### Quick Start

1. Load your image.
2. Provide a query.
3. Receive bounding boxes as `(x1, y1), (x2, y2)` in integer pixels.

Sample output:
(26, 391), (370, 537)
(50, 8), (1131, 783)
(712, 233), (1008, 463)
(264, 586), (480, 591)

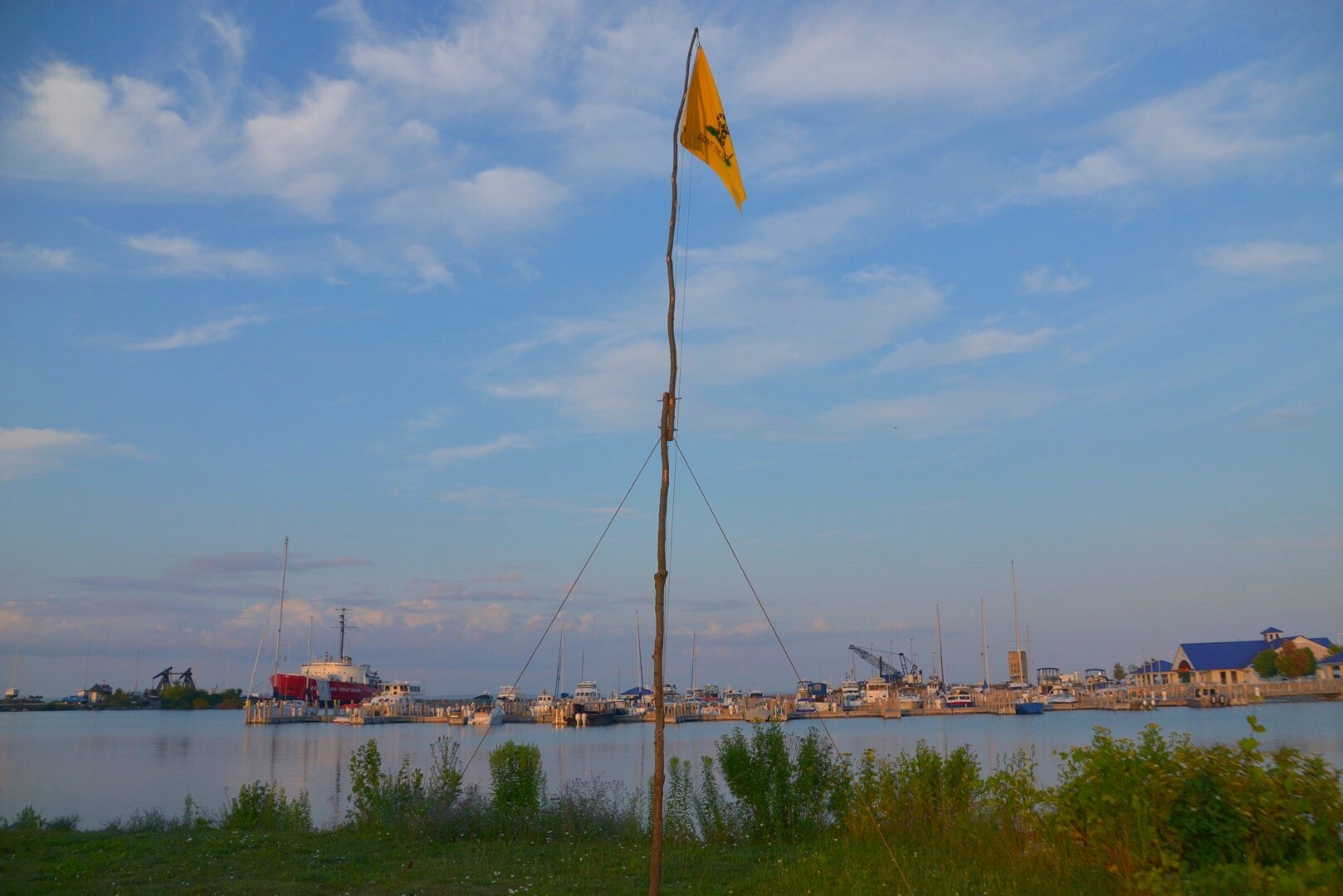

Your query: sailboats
(242, 537), (992, 728)
(1005, 558), (1046, 716)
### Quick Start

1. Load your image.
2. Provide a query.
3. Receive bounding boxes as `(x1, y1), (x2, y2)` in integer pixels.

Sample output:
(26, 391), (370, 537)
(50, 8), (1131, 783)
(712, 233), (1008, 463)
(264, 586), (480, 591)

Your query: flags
(680, 44), (746, 211)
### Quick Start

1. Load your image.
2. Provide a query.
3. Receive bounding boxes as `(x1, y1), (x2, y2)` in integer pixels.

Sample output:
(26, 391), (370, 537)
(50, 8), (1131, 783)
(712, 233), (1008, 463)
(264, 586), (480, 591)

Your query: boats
(1045, 688), (1078, 704)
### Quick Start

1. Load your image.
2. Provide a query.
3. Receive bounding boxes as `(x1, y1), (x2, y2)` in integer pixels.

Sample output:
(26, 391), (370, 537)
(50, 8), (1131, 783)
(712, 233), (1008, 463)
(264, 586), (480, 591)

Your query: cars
(60, 695), (88, 705)
